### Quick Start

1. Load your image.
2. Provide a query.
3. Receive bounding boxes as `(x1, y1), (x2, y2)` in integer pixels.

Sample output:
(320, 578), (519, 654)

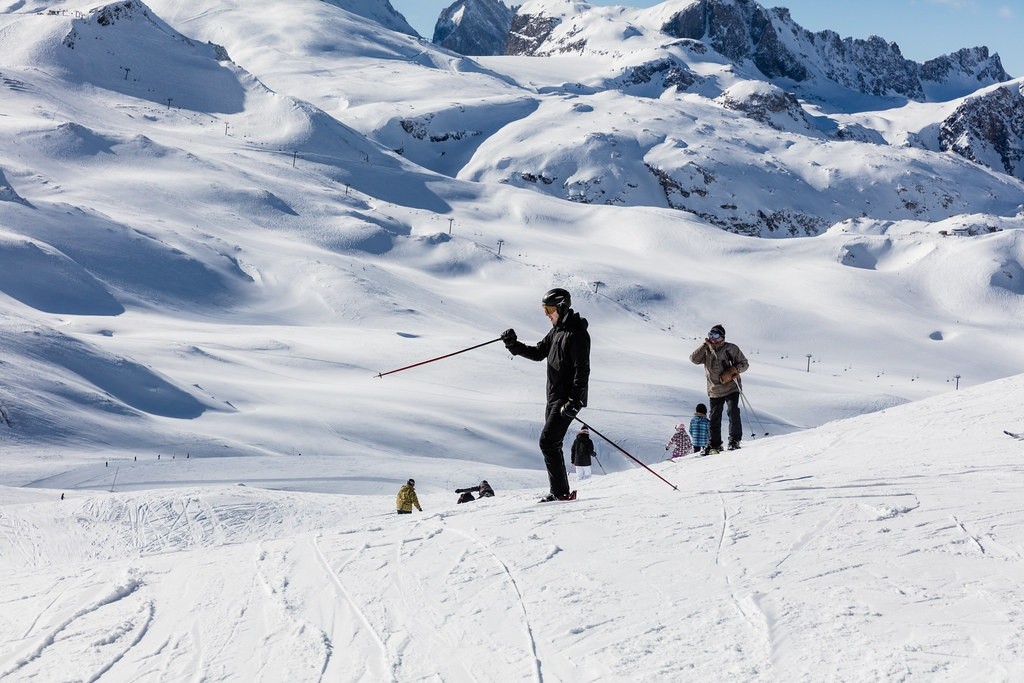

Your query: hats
(710, 325), (726, 338)
(696, 404), (707, 415)
(675, 423), (685, 430)
(581, 426), (588, 430)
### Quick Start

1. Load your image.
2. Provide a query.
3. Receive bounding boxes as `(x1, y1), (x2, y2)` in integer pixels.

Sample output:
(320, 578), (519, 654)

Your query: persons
(571, 425), (598, 480)
(689, 325), (749, 454)
(455, 480), (495, 500)
(665, 423), (693, 459)
(457, 491), (475, 504)
(396, 479), (423, 515)
(689, 403), (711, 453)
(500, 287), (592, 505)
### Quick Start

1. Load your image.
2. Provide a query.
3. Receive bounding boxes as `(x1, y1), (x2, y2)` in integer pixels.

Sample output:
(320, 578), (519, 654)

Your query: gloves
(592, 452), (596, 457)
(559, 401), (581, 420)
(571, 460), (574, 464)
(704, 338), (713, 349)
(501, 329), (518, 346)
(719, 366), (738, 385)
(666, 448), (668, 450)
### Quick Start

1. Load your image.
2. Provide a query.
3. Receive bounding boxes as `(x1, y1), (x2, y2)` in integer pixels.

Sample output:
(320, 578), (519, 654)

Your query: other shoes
(700, 443), (724, 455)
(540, 494), (569, 502)
(728, 439), (740, 450)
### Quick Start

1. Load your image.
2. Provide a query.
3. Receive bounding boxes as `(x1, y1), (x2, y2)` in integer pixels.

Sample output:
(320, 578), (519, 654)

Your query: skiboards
(665, 444), (748, 464)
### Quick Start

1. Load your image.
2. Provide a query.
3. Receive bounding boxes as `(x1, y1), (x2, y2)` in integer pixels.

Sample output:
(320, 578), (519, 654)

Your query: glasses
(708, 332), (720, 339)
(544, 306), (557, 314)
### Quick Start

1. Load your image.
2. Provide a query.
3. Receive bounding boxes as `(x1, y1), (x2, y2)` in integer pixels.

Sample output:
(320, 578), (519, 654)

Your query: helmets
(542, 288), (571, 307)
(406, 479), (415, 486)
